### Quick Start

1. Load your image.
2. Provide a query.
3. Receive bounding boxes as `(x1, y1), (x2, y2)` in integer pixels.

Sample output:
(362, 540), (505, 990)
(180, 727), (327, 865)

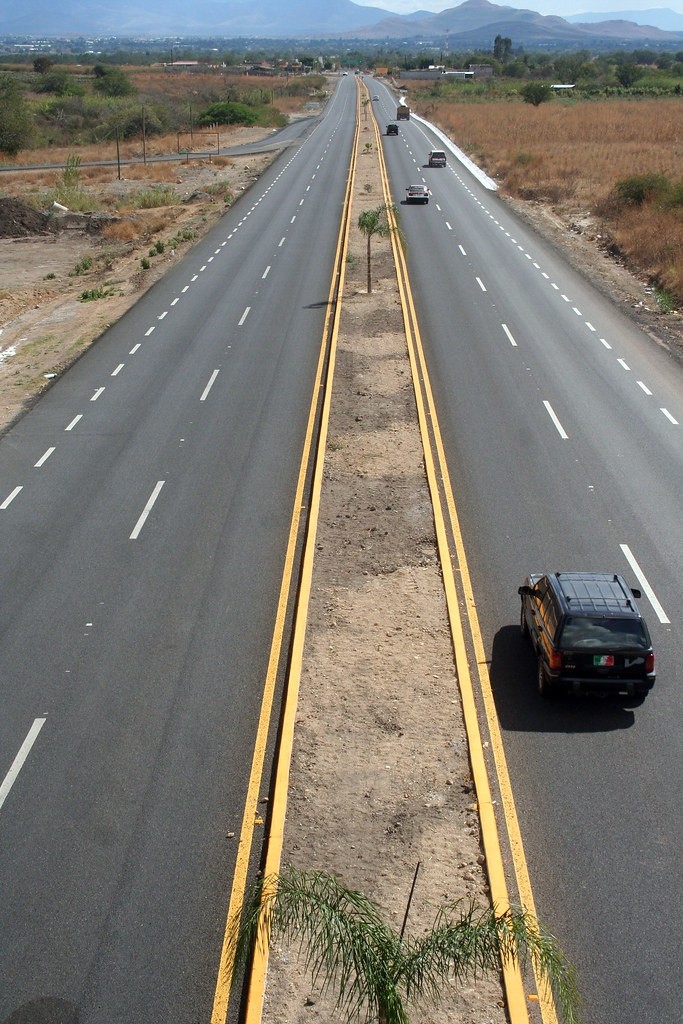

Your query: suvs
(518, 571), (656, 699)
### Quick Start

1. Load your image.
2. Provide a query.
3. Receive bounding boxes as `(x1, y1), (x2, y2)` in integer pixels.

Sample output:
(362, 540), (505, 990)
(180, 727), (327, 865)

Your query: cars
(405, 185), (430, 204)
(373, 95), (379, 101)
(386, 124), (399, 136)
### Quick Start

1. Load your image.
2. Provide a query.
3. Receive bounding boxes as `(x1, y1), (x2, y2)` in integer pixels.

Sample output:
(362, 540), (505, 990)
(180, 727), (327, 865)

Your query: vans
(428, 150), (447, 168)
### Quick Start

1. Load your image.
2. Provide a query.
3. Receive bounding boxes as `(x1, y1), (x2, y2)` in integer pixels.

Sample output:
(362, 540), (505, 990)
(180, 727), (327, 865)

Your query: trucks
(397, 105), (410, 120)
